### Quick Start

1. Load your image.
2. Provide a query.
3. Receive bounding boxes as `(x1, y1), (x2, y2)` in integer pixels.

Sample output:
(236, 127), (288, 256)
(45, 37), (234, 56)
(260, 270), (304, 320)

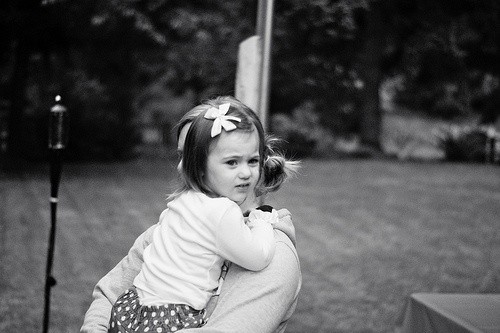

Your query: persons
(108, 95), (279, 332)
(79, 95), (303, 333)
